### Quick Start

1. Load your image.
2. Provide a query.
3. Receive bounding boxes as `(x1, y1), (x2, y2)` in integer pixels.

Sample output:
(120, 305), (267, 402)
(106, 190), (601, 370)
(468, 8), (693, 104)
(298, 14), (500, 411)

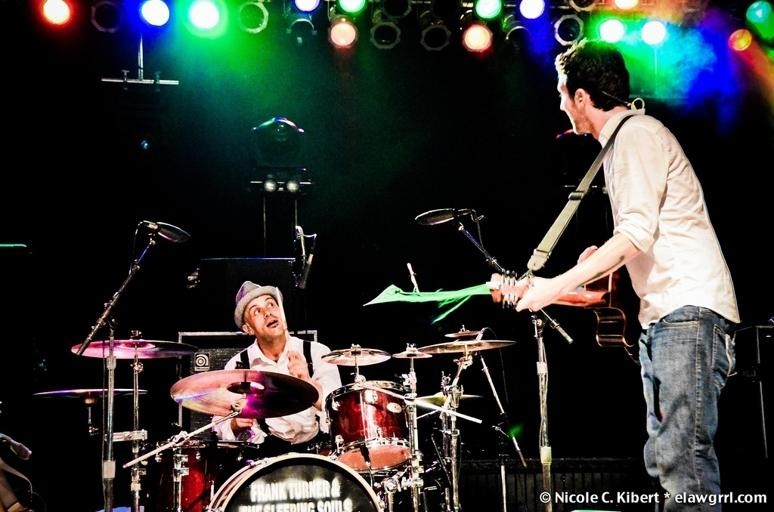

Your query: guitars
(489, 244), (643, 365)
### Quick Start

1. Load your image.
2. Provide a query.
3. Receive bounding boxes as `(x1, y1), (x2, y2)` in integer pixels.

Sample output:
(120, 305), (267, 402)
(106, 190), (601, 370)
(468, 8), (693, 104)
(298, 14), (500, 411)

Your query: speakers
(716, 319), (774, 512)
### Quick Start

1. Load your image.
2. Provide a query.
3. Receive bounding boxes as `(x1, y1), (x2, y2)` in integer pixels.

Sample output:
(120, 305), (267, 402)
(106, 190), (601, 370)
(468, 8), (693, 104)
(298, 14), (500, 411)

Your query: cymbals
(70, 338), (198, 360)
(170, 369), (319, 417)
(418, 340), (517, 354)
(444, 330), (479, 338)
(392, 350), (433, 360)
(321, 348), (393, 367)
(33, 387), (149, 401)
(415, 391), (483, 405)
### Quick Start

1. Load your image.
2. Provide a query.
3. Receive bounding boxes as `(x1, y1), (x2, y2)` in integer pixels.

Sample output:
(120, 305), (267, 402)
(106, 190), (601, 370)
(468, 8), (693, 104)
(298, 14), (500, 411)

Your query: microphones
(0, 432), (33, 461)
(415, 207), (471, 227)
(154, 221), (193, 244)
(294, 252), (314, 291)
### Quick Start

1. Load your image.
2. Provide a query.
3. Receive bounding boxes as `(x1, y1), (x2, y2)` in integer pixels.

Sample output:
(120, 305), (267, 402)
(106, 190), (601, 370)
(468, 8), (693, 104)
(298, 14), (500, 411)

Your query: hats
(235, 280), (283, 328)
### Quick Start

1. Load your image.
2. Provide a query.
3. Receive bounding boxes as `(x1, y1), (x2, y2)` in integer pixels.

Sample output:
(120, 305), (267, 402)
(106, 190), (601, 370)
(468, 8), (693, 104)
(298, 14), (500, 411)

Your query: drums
(207, 452), (386, 512)
(325, 380), (412, 473)
(169, 442), (257, 512)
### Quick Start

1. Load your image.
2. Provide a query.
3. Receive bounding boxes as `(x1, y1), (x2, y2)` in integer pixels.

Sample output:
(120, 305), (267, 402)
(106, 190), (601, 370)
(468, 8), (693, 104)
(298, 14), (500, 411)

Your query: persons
(207, 277), (347, 495)
(512, 35), (740, 511)
